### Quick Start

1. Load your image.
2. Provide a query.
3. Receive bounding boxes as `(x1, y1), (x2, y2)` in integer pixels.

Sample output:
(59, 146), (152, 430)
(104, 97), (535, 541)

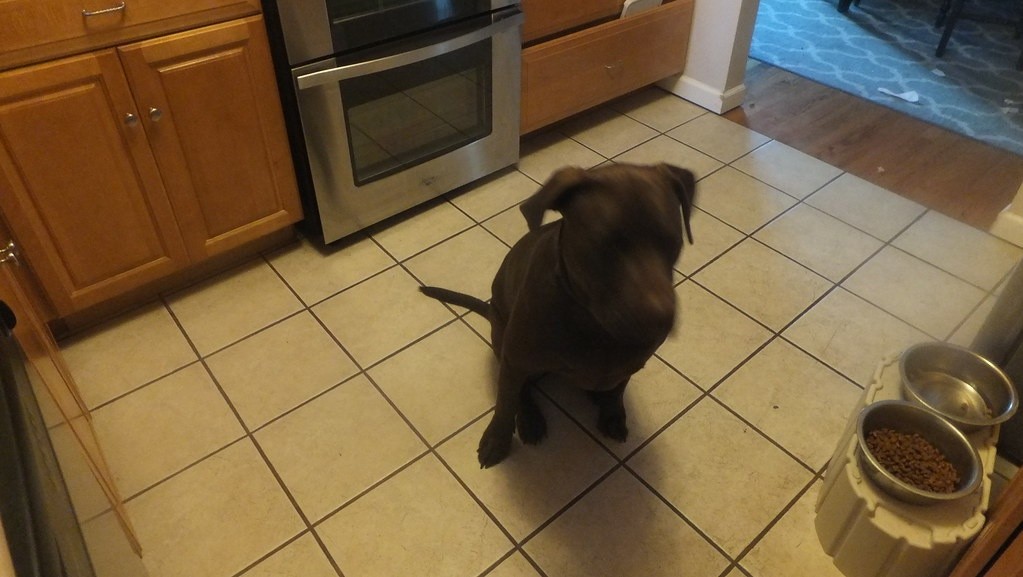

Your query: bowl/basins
(900, 343), (1018, 432)
(855, 400), (983, 502)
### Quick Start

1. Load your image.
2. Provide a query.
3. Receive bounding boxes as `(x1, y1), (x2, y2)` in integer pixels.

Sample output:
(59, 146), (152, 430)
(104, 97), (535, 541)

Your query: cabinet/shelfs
(519, 0), (694, 135)
(0, 0), (305, 325)
(1, 244), (154, 577)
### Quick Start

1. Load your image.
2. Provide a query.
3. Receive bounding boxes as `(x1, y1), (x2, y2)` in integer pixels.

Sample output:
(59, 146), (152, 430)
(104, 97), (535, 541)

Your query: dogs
(417, 162), (699, 470)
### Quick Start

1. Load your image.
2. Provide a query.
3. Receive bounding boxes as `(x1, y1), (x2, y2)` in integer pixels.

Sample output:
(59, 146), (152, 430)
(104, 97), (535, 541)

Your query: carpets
(747, 0), (1023, 160)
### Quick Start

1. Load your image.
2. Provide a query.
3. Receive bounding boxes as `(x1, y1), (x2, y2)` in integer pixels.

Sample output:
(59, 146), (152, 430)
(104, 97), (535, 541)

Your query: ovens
(259, 0), (521, 247)
